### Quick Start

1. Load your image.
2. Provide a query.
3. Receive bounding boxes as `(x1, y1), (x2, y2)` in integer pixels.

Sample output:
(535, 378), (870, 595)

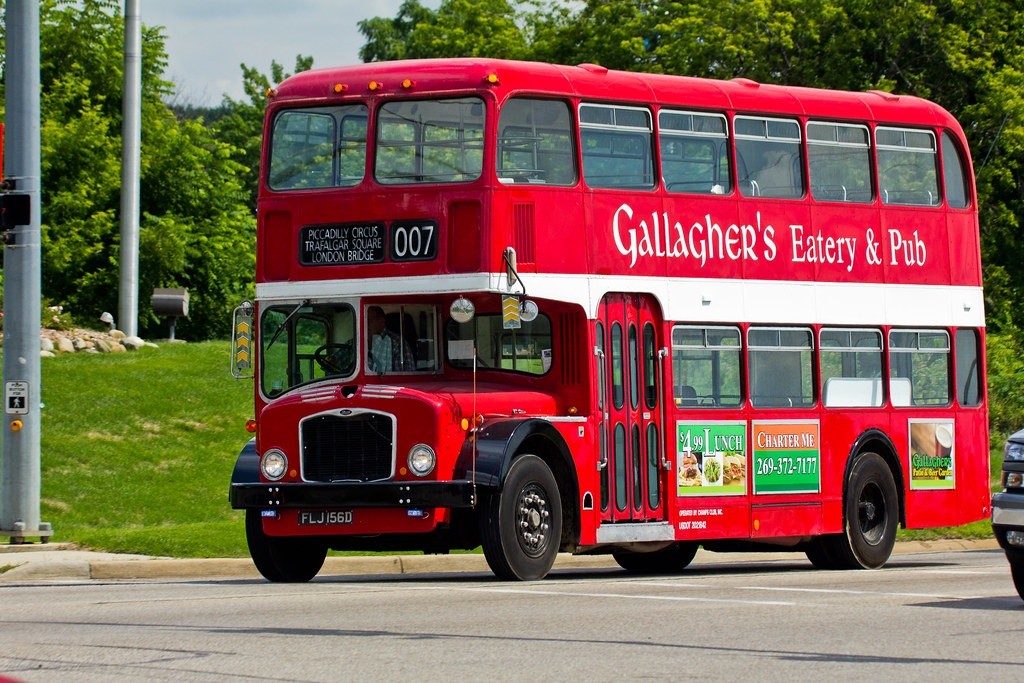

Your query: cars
(990, 425), (1024, 600)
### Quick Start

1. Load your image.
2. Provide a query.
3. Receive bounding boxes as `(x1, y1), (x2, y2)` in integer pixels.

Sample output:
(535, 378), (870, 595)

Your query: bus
(229, 55), (994, 573)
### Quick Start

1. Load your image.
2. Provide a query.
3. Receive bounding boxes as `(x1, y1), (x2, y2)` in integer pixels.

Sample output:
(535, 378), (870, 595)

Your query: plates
(701, 451), (724, 488)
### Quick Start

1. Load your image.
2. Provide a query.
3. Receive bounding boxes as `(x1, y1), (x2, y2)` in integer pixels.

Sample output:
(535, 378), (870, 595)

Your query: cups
(934, 426), (952, 480)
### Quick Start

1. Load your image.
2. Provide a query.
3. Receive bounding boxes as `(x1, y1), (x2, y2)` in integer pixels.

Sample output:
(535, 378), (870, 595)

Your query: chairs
(323, 172), (943, 408)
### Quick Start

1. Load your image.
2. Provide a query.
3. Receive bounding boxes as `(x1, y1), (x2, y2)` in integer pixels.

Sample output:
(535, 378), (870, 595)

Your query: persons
(320, 305), (416, 374)
